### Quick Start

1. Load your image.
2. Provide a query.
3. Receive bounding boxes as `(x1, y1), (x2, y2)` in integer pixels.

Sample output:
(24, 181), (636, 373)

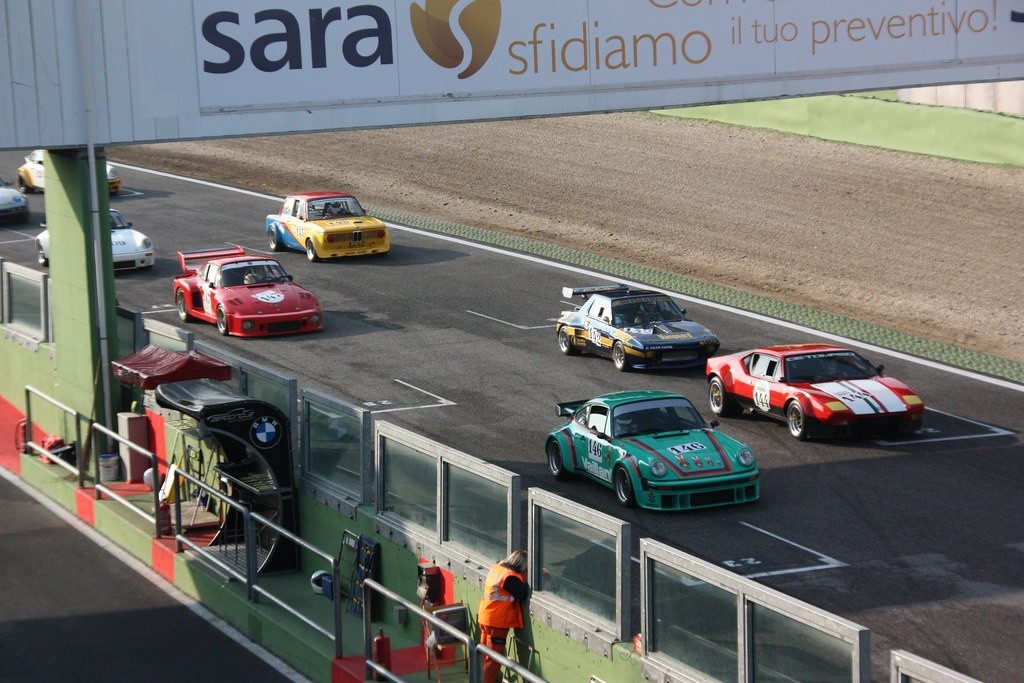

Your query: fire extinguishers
(159, 499), (171, 535)
(374, 627), (391, 681)
(14, 418), (32, 453)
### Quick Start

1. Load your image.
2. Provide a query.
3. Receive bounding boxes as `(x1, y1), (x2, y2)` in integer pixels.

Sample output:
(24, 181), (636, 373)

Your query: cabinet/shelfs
(117, 412), (149, 484)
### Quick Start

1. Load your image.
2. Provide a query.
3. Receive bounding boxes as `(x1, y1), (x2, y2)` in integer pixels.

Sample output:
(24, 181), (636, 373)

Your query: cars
(0, 179), (28, 226)
(554, 282), (721, 372)
(34, 208), (155, 275)
(15, 148), (120, 197)
(265, 190), (391, 262)
(172, 246), (325, 338)
(704, 343), (925, 441)
(543, 389), (762, 514)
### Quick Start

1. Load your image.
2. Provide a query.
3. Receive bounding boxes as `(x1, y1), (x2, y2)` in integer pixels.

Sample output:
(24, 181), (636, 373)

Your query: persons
(325, 201), (349, 219)
(476, 549), (530, 683)
(244, 266), (276, 286)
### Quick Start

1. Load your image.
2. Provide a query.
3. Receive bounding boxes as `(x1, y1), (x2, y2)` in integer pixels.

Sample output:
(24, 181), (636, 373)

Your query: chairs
(422, 599), (469, 683)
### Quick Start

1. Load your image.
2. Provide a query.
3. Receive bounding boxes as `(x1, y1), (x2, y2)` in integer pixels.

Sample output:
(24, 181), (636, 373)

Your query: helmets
(329, 201), (341, 212)
(311, 570), (332, 594)
(821, 357), (839, 370)
(640, 301), (656, 312)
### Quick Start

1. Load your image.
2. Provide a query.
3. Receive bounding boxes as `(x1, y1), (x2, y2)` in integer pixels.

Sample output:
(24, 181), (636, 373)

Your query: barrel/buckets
(98, 454), (118, 481)
